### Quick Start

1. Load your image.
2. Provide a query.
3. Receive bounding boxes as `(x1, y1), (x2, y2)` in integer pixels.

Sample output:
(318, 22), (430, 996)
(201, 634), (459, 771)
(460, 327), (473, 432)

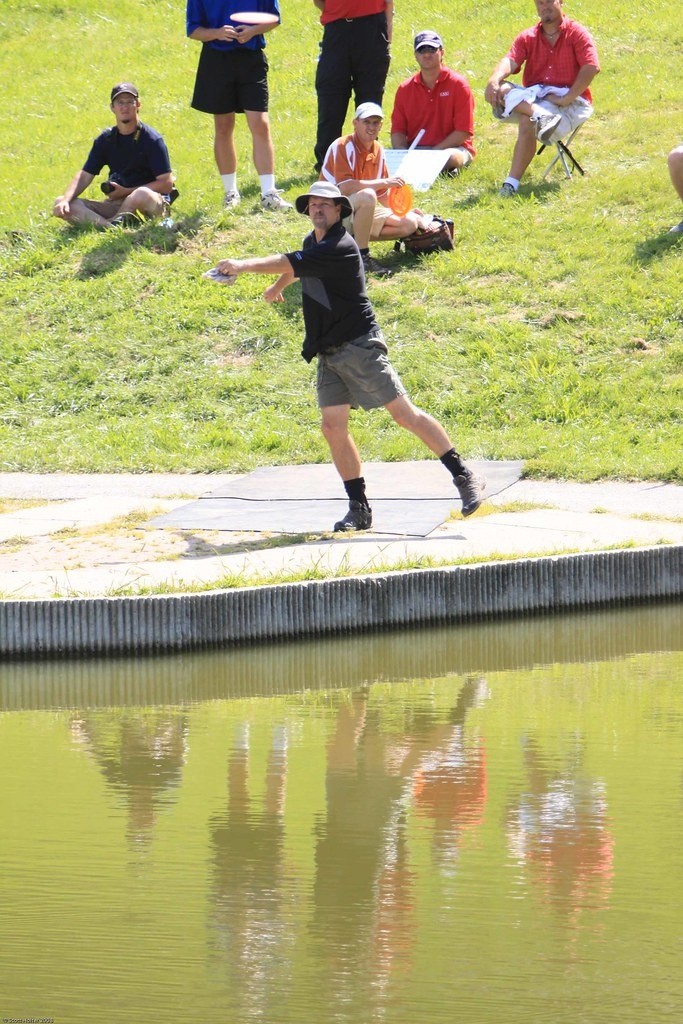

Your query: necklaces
(543, 29), (559, 38)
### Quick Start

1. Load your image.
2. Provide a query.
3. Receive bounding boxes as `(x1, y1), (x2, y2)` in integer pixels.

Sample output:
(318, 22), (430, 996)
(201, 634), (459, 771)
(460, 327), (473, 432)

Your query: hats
(110, 82), (139, 102)
(294, 182), (353, 220)
(414, 31), (443, 52)
(354, 102), (383, 120)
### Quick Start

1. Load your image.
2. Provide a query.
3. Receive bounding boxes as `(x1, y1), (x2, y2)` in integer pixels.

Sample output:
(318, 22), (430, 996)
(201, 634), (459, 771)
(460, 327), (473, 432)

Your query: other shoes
(448, 167), (460, 178)
(670, 219), (683, 233)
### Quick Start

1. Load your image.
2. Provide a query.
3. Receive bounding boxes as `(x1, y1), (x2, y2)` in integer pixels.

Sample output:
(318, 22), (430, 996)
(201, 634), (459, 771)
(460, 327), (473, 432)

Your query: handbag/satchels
(405, 215), (456, 256)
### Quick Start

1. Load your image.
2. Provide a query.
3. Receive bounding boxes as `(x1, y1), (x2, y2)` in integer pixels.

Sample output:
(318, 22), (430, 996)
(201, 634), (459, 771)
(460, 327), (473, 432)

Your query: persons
(313, 0), (393, 173)
(485, 0), (601, 198)
(391, 31), (476, 174)
(185, 0), (295, 214)
(318, 102), (424, 277)
(53, 83), (175, 233)
(215, 182), (487, 533)
(667, 144), (683, 232)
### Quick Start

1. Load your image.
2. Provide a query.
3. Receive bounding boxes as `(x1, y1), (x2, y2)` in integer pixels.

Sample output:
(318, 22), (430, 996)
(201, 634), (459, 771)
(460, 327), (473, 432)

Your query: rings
(224, 269), (228, 273)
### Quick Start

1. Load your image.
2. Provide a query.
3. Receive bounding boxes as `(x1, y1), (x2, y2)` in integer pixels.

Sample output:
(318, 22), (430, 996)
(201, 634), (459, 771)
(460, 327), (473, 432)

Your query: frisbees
(229, 12), (278, 25)
(388, 184), (412, 216)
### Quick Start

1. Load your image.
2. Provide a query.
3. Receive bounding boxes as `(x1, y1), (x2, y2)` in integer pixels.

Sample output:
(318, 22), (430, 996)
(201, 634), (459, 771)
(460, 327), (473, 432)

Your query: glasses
(417, 45), (438, 54)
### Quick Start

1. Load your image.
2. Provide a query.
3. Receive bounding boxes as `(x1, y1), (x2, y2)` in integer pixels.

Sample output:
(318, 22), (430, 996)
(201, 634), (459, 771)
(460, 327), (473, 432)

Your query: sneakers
(529, 113), (563, 143)
(334, 500), (372, 532)
(453, 470), (487, 517)
(497, 183), (515, 199)
(223, 191), (241, 213)
(361, 254), (391, 279)
(259, 189), (293, 213)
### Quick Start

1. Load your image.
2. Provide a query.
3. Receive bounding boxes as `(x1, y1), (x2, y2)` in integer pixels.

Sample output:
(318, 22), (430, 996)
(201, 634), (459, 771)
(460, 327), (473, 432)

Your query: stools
(542, 123), (583, 181)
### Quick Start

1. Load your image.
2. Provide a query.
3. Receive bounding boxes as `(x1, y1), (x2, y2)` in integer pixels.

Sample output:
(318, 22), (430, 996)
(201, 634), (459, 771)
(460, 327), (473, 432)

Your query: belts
(321, 343), (343, 355)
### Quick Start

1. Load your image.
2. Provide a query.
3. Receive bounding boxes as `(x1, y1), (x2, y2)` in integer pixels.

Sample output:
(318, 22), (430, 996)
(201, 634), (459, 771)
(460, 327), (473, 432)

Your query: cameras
(100, 172), (124, 194)
